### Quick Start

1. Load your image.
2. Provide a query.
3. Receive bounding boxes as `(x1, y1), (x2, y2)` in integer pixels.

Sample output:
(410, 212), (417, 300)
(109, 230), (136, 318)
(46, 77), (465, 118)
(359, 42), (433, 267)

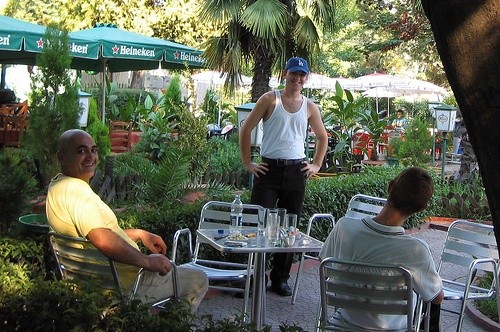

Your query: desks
(110, 131), (178, 153)
(196, 226), (325, 332)
(0, 127), (25, 149)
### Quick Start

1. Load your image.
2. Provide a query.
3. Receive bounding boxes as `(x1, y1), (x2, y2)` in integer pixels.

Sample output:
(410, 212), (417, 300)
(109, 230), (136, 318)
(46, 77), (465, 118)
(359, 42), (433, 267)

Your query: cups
(257, 207), (297, 243)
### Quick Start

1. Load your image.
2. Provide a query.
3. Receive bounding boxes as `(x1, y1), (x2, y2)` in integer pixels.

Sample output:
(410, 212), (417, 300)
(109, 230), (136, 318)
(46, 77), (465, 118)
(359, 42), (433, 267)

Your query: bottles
(229, 195), (243, 234)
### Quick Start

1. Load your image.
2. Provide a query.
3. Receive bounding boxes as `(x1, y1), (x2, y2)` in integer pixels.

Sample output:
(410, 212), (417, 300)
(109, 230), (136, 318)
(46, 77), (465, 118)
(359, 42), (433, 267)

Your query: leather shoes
(272, 281), (292, 296)
(235, 284), (267, 298)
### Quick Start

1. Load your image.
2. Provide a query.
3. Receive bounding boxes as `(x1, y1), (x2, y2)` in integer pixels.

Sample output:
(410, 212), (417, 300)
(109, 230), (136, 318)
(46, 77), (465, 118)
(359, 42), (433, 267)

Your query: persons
(319, 166), (444, 332)
(45, 128), (209, 324)
(234, 57), (328, 299)
(390, 109), (409, 139)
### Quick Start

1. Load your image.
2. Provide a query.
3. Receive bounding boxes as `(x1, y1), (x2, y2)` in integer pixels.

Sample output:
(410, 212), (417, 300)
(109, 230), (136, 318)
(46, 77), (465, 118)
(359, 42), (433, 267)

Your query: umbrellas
(190, 72), (449, 128)
(0, 15), (209, 126)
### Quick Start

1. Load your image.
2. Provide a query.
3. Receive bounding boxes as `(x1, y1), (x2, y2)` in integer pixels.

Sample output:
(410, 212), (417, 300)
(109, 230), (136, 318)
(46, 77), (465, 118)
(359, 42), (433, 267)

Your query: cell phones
(224, 241), (248, 246)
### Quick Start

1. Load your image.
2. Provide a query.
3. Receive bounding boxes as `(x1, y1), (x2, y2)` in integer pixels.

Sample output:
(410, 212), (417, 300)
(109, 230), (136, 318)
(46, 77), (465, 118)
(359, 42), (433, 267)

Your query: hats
(0, 89), (16, 104)
(285, 57), (309, 75)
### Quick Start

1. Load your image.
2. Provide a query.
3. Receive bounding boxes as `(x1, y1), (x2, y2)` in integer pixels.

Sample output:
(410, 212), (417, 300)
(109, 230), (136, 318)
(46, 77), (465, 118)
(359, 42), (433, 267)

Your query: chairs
(2, 100), (28, 147)
(19, 193), (500, 332)
(207, 119), (235, 141)
(109, 120), (132, 153)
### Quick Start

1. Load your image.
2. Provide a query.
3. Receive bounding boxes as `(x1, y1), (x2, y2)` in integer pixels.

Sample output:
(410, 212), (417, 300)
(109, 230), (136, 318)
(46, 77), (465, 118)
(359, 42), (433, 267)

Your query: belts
(261, 157), (305, 167)
